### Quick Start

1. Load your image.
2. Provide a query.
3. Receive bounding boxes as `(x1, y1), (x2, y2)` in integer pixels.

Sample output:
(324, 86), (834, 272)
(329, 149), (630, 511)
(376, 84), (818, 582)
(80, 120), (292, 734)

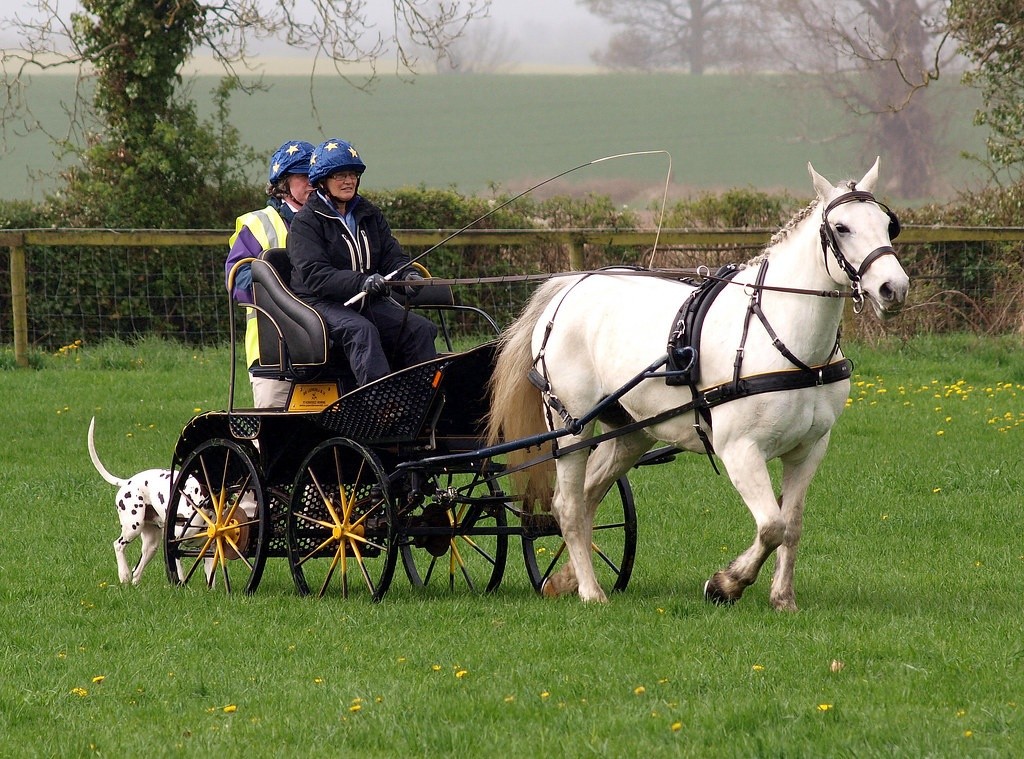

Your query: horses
(472, 156), (911, 611)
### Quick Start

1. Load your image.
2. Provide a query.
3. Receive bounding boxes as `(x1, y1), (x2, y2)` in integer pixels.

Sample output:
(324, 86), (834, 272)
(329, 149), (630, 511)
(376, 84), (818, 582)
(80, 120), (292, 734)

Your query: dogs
(88, 416), (256, 591)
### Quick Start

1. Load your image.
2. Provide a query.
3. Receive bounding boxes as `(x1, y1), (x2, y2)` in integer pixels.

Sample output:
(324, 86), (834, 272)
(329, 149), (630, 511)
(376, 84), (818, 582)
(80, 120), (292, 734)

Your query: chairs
(250, 246), (455, 378)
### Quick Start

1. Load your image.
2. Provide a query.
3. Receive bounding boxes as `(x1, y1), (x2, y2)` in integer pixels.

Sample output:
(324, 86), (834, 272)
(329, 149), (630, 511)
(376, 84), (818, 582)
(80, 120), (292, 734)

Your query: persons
(224, 141), (318, 548)
(286, 138), (437, 436)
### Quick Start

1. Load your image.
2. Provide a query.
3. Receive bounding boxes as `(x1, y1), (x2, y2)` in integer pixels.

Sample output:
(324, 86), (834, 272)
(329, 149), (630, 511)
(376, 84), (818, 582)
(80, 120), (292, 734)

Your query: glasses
(327, 173), (360, 181)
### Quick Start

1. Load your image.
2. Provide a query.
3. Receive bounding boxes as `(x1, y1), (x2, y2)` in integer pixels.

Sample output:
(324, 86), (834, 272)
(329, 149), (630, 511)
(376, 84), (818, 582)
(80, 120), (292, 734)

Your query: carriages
(164, 148), (918, 612)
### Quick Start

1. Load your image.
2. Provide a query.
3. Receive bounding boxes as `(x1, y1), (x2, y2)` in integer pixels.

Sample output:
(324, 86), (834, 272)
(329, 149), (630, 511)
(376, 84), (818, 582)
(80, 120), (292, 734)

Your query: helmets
(309, 138), (367, 190)
(269, 140), (316, 182)
